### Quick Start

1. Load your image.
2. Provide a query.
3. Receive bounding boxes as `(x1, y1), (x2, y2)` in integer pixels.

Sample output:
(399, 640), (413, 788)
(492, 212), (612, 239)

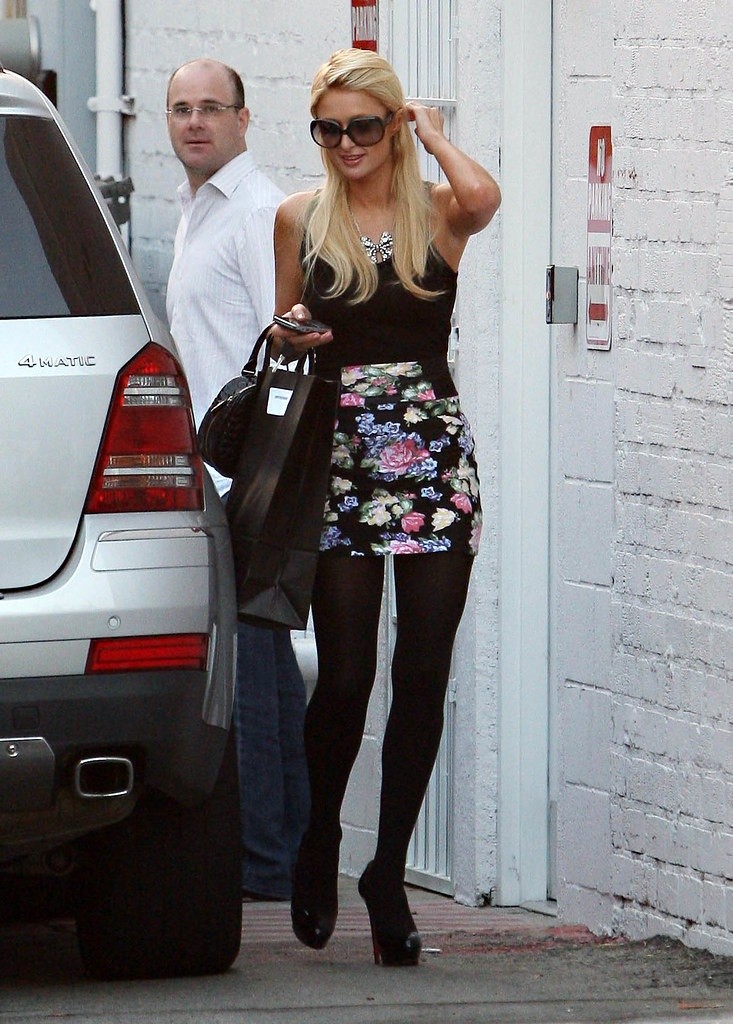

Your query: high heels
(291, 827), (342, 949)
(358, 860), (421, 966)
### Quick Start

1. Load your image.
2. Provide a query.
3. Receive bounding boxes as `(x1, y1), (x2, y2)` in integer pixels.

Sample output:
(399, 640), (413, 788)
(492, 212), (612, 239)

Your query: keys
(272, 340), (296, 374)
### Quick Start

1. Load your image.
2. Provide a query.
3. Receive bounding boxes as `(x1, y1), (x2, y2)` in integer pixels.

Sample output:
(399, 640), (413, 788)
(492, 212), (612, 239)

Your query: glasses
(310, 112), (393, 148)
(165, 104), (240, 118)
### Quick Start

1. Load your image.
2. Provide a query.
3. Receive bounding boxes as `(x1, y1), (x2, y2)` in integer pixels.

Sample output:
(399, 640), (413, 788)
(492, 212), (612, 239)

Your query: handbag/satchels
(226, 327), (342, 632)
(197, 322), (315, 479)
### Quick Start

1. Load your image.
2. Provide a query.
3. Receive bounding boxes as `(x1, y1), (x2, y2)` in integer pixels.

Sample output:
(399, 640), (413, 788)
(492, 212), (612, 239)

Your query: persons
(164, 59), (307, 903)
(197, 48), (501, 965)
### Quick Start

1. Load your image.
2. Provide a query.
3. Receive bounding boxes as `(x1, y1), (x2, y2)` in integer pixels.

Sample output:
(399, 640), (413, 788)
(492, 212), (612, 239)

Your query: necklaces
(349, 200), (397, 263)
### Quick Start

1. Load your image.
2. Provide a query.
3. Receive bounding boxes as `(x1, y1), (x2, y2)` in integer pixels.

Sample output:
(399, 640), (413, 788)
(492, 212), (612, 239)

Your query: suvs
(1, 71), (249, 986)
